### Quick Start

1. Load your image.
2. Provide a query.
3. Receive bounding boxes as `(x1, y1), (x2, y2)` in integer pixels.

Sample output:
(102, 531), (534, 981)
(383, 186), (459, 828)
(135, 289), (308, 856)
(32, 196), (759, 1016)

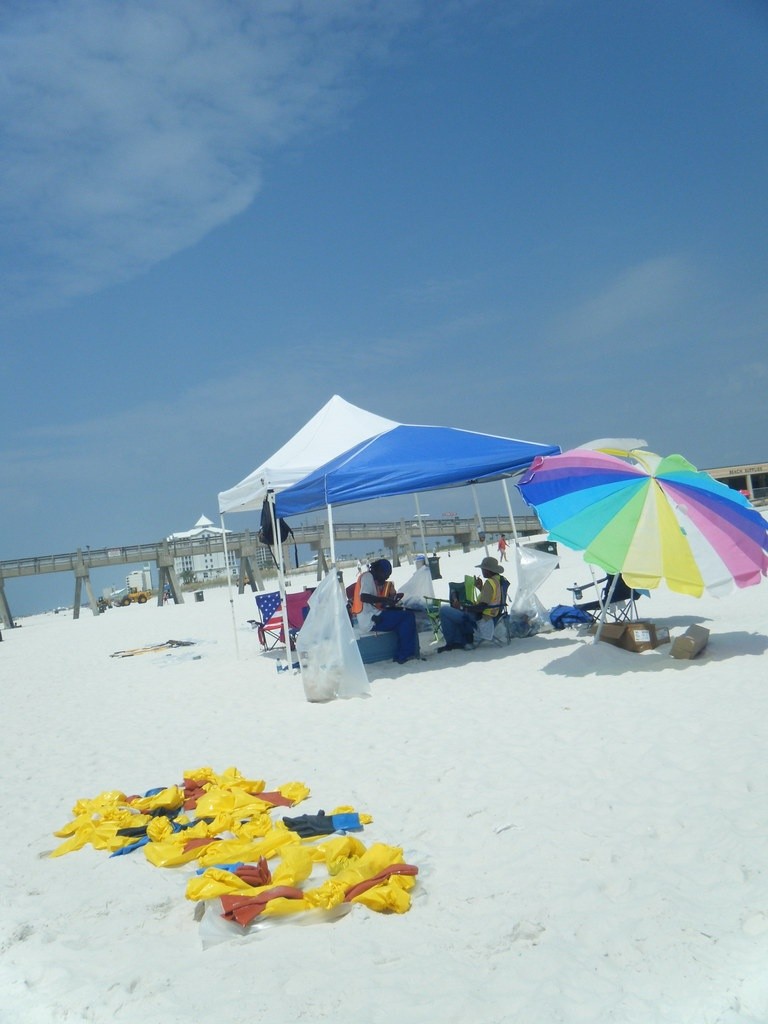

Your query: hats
(475, 557), (504, 573)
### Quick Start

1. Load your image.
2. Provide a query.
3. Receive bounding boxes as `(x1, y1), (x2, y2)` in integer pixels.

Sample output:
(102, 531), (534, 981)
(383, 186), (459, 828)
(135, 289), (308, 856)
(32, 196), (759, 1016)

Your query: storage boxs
(587, 622), (658, 654)
(668, 623), (710, 659)
(655, 627), (671, 645)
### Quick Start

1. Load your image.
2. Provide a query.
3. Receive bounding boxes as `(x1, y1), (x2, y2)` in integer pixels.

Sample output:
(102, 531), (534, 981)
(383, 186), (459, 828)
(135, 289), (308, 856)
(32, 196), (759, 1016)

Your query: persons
(436, 556), (504, 654)
(433, 545), (436, 556)
(366, 557), (370, 572)
(354, 558), (363, 577)
(352, 558), (426, 665)
(497, 534), (510, 562)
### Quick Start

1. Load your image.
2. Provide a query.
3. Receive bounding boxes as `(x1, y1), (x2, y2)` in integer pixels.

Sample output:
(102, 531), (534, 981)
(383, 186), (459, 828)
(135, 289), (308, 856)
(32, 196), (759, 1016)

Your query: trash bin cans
(415, 556), (443, 580)
(194, 591), (204, 603)
(523, 541), (559, 569)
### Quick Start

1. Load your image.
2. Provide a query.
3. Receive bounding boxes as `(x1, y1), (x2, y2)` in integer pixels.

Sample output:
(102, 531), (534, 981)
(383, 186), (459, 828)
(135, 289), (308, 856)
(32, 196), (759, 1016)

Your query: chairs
(469, 575), (510, 649)
(255, 592), (290, 652)
(285, 591), (312, 642)
(422, 575), (476, 645)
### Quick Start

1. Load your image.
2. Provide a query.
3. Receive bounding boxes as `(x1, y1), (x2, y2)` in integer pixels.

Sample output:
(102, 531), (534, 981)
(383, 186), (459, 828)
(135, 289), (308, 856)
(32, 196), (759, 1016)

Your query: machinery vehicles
(109, 586), (151, 606)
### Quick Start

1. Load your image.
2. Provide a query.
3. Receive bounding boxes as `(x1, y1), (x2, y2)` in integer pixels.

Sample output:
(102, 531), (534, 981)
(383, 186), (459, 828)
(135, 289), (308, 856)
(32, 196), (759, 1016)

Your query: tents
(213, 393), (562, 680)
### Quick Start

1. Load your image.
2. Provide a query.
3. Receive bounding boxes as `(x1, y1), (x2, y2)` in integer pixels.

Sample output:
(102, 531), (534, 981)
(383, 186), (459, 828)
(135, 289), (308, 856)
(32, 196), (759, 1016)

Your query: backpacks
(549, 604), (596, 630)
(257, 490), (301, 572)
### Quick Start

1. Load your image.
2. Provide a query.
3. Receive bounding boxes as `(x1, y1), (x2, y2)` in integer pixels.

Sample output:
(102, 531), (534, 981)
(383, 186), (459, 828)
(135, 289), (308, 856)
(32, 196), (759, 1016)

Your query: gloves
(47, 762), (418, 933)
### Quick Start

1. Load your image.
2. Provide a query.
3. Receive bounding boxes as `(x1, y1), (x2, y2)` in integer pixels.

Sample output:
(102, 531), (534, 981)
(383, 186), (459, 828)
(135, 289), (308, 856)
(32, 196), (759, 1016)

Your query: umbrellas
(513, 436), (767, 646)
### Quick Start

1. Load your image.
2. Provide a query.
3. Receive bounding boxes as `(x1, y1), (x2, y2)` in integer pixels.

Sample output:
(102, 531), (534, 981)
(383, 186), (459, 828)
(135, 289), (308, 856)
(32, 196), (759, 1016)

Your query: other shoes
(393, 655), (427, 664)
(437, 642), (466, 653)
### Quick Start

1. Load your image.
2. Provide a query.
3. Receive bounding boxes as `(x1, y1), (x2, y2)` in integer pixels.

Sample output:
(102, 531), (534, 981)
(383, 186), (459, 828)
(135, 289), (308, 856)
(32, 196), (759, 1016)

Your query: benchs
(574, 573), (640, 629)
(353, 630), (418, 664)
